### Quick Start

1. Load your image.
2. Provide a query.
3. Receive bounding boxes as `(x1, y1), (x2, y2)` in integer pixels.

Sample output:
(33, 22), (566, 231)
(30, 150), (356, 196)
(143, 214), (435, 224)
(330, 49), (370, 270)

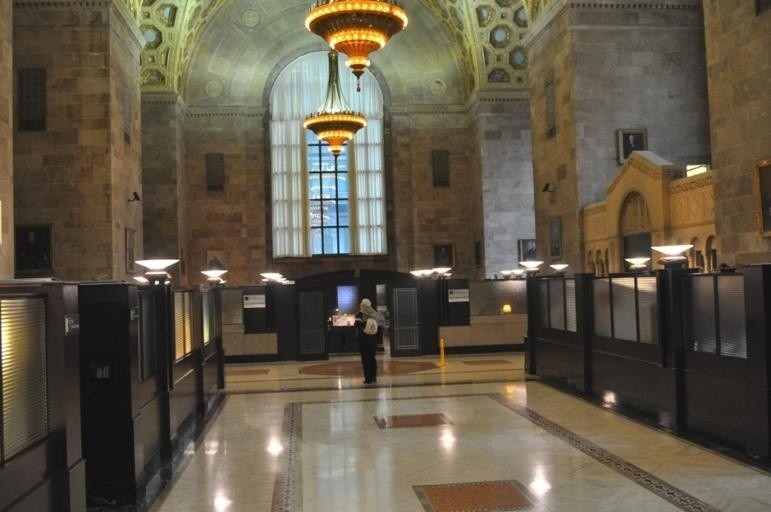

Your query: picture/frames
(750, 158), (771, 238)
(204, 247), (228, 271)
(517, 238), (538, 264)
(432, 242), (457, 269)
(546, 215), (564, 261)
(124, 225), (137, 275)
(615, 127), (648, 166)
(14, 222), (54, 276)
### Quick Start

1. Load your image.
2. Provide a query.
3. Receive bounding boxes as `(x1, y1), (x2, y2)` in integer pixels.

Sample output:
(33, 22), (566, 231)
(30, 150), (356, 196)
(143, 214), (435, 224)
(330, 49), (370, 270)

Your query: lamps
(297, 2), (410, 168)
(500, 262), (569, 281)
(409, 267), (452, 281)
(494, 302), (512, 315)
(135, 246), (230, 282)
(257, 269), (296, 286)
(541, 183), (555, 193)
(625, 239), (694, 267)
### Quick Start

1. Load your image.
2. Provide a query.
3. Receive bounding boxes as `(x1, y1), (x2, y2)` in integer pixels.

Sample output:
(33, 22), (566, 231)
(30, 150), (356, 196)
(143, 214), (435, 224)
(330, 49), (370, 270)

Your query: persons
(624, 134), (641, 159)
(354, 296), (386, 385)
(17, 230), (47, 269)
(528, 242), (536, 258)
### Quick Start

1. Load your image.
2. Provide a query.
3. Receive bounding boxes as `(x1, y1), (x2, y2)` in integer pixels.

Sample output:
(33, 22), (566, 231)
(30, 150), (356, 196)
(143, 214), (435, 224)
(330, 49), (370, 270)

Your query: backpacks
(355, 318), (378, 335)
